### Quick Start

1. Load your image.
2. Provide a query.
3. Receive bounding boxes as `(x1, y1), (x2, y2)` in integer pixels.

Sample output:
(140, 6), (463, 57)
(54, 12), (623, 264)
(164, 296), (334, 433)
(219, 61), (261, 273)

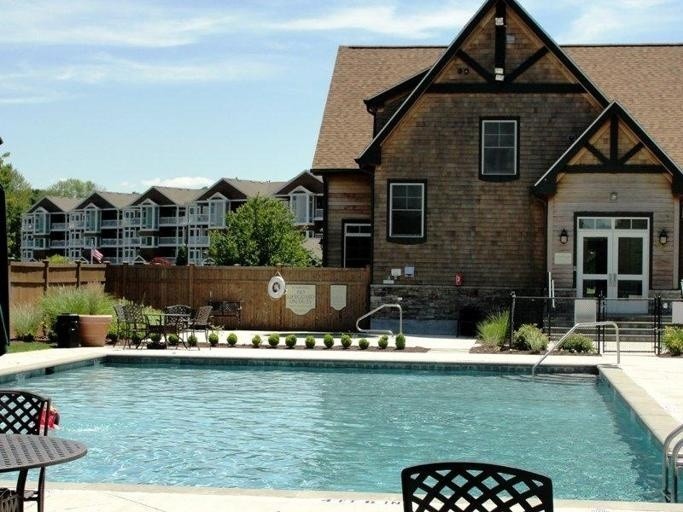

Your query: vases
(78, 314), (113, 346)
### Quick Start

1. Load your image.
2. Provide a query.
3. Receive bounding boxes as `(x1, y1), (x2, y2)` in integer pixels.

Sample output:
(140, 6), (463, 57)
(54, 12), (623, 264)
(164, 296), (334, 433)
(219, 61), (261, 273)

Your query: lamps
(659, 229), (667, 245)
(560, 228), (568, 244)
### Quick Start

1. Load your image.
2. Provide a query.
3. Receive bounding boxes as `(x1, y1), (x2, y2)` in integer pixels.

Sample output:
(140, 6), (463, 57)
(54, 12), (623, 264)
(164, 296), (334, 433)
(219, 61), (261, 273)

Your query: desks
(0, 433), (88, 512)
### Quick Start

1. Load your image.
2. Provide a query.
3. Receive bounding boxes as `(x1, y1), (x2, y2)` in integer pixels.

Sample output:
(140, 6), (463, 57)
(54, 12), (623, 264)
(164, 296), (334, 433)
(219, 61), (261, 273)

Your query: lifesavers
(267, 277), (285, 298)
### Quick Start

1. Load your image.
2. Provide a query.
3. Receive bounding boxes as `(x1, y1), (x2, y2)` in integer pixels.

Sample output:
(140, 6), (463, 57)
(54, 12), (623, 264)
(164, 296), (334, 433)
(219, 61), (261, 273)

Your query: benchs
(209, 301), (241, 330)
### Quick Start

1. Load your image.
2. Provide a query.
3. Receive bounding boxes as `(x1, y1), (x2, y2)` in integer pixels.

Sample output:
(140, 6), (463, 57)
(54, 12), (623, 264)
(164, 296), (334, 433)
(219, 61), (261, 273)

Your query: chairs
(401, 460), (553, 512)
(113, 303), (212, 350)
(0, 389), (51, 512)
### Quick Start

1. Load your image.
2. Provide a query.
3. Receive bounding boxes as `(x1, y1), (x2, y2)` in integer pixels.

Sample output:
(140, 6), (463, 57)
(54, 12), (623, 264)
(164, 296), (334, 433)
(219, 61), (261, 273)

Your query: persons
(36, 404), (60, 428)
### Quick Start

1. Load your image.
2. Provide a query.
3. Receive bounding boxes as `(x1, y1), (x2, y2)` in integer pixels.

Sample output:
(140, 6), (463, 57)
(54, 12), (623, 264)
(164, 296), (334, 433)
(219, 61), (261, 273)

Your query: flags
(92, 247), (103, 263)
